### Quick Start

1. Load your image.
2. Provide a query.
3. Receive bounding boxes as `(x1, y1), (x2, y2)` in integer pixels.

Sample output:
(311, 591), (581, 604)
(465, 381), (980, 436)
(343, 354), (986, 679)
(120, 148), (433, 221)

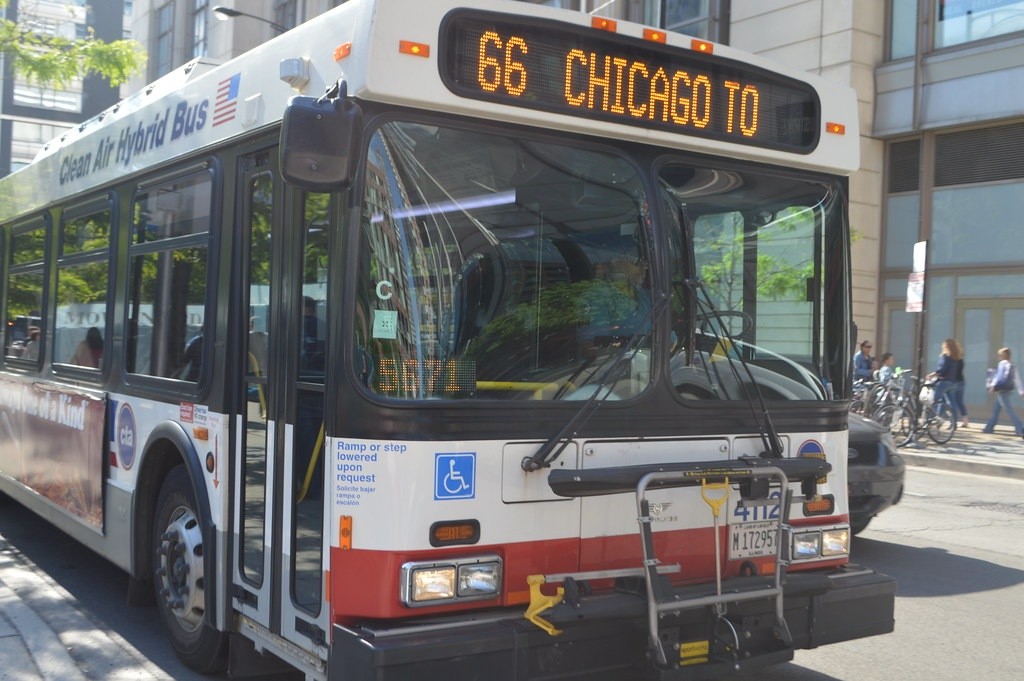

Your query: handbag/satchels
(918, 379), (935, 404)
(985, 364), (1015, 391)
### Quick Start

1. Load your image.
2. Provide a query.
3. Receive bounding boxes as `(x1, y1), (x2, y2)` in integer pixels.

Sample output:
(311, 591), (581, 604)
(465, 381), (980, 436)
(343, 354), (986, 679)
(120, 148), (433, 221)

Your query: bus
(0, 0), (896, 681)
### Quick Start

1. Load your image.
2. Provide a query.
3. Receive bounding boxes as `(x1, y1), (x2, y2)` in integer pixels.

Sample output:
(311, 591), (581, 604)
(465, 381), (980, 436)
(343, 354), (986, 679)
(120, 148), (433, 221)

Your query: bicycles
(849, 368), (957, 448)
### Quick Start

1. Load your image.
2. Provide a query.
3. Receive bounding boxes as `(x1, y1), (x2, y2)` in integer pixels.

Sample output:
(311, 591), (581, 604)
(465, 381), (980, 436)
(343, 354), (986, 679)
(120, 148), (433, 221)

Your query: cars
(848, 412), (907, 536)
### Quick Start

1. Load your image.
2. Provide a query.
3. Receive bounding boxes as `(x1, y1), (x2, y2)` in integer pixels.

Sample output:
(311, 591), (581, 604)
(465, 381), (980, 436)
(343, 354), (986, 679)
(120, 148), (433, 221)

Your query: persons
(982, 347), (1024, 436)
(574, 232), (679, 357)
(944, 343), (969, 430)
(877, 353), (901, 432)
(69, 326), (105, 370)
(15, 324), (50, 361)
(923, 338), (960, 432)
(176, 292), (329, 390)
(853, 340), (875, 394)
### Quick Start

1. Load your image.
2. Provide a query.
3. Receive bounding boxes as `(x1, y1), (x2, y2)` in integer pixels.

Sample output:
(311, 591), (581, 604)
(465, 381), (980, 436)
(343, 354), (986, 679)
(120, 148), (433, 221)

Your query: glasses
(864, 346), (872, 348)
(623, 254), (649, 269)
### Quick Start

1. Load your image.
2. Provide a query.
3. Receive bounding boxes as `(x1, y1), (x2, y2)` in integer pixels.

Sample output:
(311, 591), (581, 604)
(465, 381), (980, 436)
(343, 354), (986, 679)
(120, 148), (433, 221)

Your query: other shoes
(946, 423), (957, 429)
(960, 424), (969, 428)
